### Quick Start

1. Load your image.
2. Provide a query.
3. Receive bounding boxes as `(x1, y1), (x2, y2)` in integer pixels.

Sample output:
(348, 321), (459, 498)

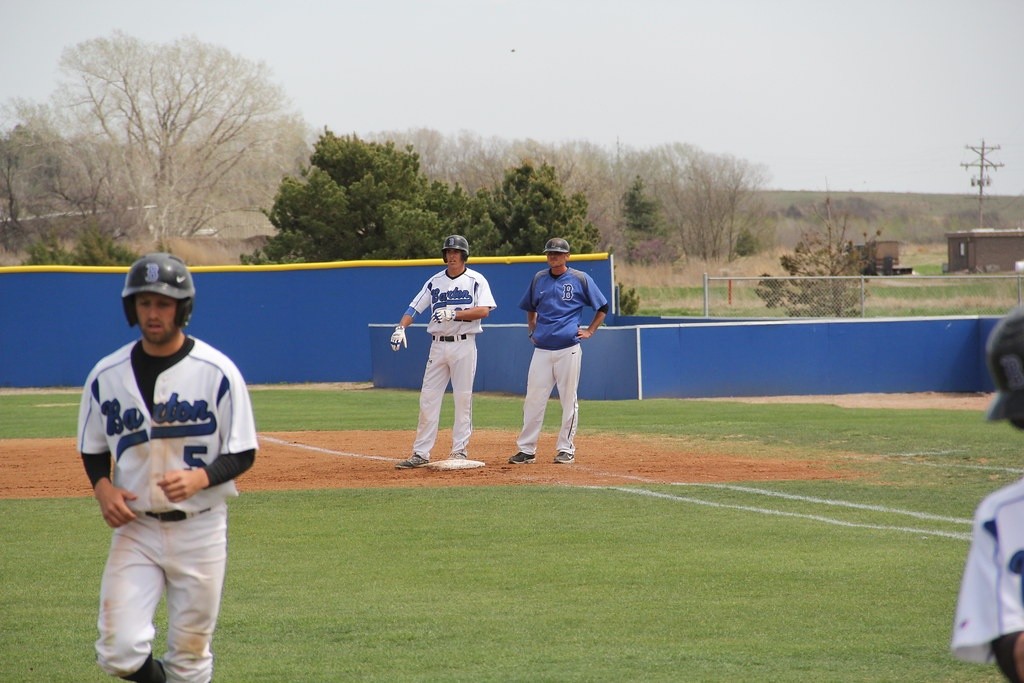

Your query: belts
(145, 509), (211, 521)
(432, 334), (467, 341)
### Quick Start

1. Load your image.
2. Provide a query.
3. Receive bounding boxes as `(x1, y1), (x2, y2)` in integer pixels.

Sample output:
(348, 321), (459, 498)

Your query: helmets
(441, 235), (469, 261)
(541, 238), (571, 253)
(120, 254), (195, 329)
(987, 302), (1023, 424)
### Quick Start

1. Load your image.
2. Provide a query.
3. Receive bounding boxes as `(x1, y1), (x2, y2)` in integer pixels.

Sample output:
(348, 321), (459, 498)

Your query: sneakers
(395, 455), (429, 468)
(447, 453), (466, 460)
(554, 451), (575, 464)
(508, 452), (536, 464)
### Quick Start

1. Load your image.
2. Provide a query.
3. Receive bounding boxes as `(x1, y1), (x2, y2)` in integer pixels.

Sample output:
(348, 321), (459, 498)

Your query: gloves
(431, 308), (456, 324)
(390, 325), (408, 351)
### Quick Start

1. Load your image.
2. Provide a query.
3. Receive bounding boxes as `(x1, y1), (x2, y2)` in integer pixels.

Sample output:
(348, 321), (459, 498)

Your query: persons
(508, 238), (609, 464)
(75, 252), (259, 683)
(951, 307), (1024, 683)
(391, 235), (497, 469)
